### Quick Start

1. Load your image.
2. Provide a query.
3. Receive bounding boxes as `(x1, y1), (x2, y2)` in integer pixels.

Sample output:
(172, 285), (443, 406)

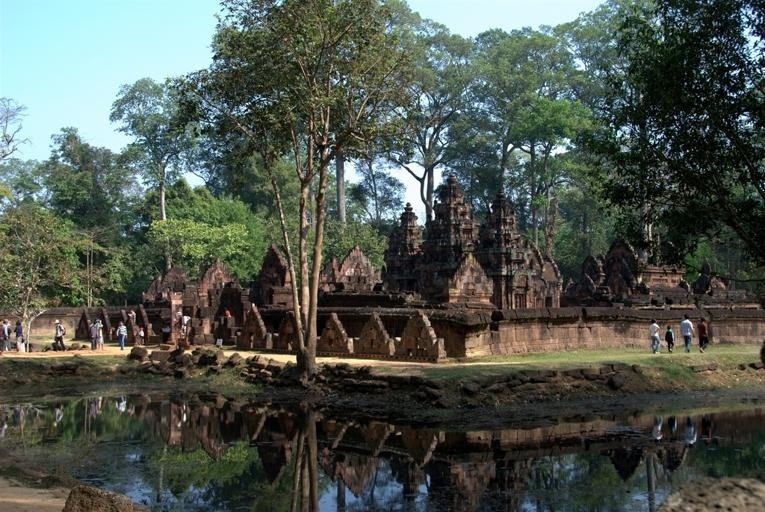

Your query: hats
(177, 312), (182, 315)
(54, 319), (60, 324)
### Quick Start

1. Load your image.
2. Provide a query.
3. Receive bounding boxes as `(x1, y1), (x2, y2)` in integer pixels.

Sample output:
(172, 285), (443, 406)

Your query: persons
(174, 311), (191, 339)
(665, 324), (675, 353)
(685, 415), (698, 449)
(701, 414), (712, 449)
(51, 402), (64, 428)
(139, 326), (145, 344)
(0, 402), (25, 439)
(680, 313), (696, 352)
(668, 415), (678, 444)
(649, 318), (661, 354)
(89, 317), (105, 351)
(697, 317), (709, 353)
(87, 394), (135, 420)
(52, 319), (67, 351)
(651, 414), (664, 446)
(162, 325), (171, 341)
(175, 402), (188, 427)
(0, 318), (26, 353)
(116, 321), (127, 350)
(223, 305), (232, 318)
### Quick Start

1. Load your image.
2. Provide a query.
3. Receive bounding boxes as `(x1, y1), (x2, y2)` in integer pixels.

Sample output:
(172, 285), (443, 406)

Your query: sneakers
(654, 349), (705, 354)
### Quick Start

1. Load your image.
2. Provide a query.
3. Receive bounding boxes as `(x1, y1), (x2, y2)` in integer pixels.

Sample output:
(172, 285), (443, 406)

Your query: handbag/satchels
(60, 325), (66, 335)
(3, 328), (12, 337)
